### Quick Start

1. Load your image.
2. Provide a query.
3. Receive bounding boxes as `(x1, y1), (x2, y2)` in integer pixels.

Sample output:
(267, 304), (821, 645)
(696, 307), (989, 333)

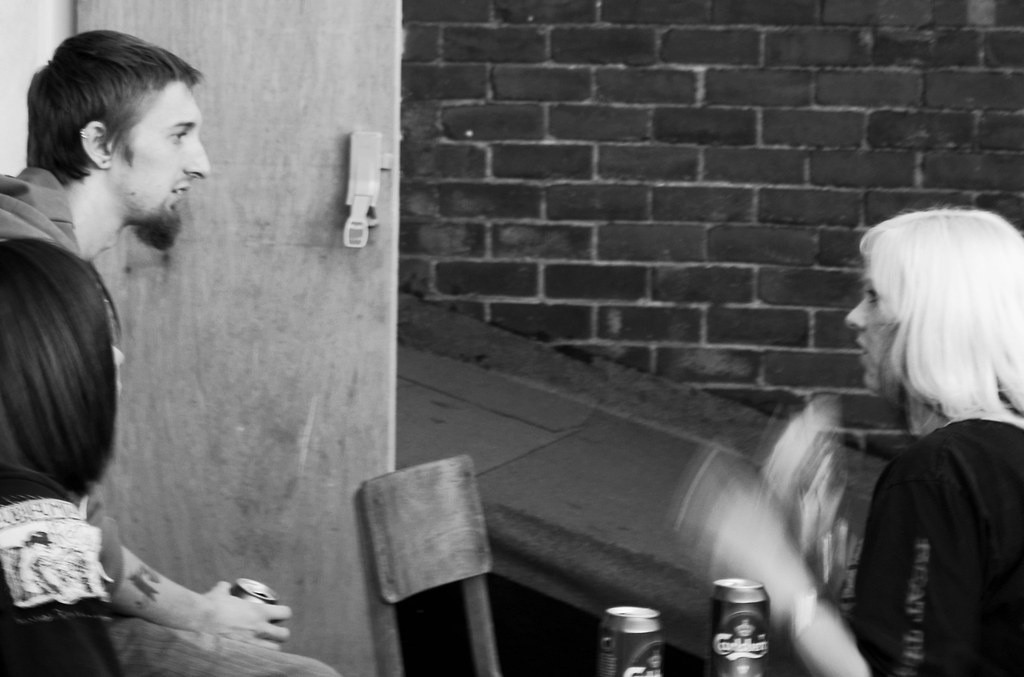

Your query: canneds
(598, 606), (663, 677)
(708, 578), (770, 677)
(229, 577), (280, 604)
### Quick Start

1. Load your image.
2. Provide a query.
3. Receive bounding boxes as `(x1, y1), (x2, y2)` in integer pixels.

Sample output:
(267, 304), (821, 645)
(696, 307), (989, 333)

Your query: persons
(0, 29), (341, 677)
(676, 199), (1024, 677)
